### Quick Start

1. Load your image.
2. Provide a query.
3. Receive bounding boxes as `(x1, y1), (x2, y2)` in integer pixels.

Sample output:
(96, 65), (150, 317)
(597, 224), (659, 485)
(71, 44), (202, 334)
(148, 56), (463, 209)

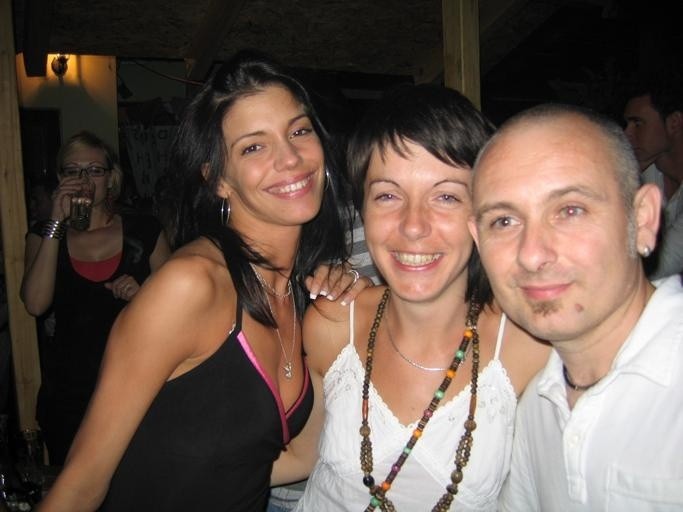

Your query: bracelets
(42, 217), (67, 241)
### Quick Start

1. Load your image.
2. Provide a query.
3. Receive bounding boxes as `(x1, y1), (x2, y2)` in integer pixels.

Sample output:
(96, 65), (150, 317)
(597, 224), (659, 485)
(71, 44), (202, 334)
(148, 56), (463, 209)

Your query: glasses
(63, 166), (113, 177)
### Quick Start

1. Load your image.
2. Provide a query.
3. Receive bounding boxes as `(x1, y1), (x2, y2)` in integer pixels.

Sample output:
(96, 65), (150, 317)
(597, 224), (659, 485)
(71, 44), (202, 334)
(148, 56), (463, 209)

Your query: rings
(348, 269), (359, 285)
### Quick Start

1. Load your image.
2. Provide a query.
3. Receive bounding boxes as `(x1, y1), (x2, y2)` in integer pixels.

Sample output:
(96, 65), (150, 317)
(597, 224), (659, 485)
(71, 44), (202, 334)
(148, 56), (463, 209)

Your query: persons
(257, 82), (552, 512)
(619, 72), (683, 278)
(18, 130), (169, 466)
(31, 46), (377, 512)
(463, 99), (683, 512)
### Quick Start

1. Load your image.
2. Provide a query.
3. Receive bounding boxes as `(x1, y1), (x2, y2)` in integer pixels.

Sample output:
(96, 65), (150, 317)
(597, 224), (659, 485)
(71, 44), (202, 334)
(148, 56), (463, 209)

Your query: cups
(70, 176), (96, 231)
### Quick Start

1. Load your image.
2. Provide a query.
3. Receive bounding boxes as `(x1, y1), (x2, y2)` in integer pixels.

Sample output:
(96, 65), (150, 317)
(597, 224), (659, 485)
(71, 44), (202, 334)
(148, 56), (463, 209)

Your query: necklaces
(562, 361), (605, 390)
(251, 267), (296, 380)
(384, 298), (472, 372)
(359, 286), (482, 512)
